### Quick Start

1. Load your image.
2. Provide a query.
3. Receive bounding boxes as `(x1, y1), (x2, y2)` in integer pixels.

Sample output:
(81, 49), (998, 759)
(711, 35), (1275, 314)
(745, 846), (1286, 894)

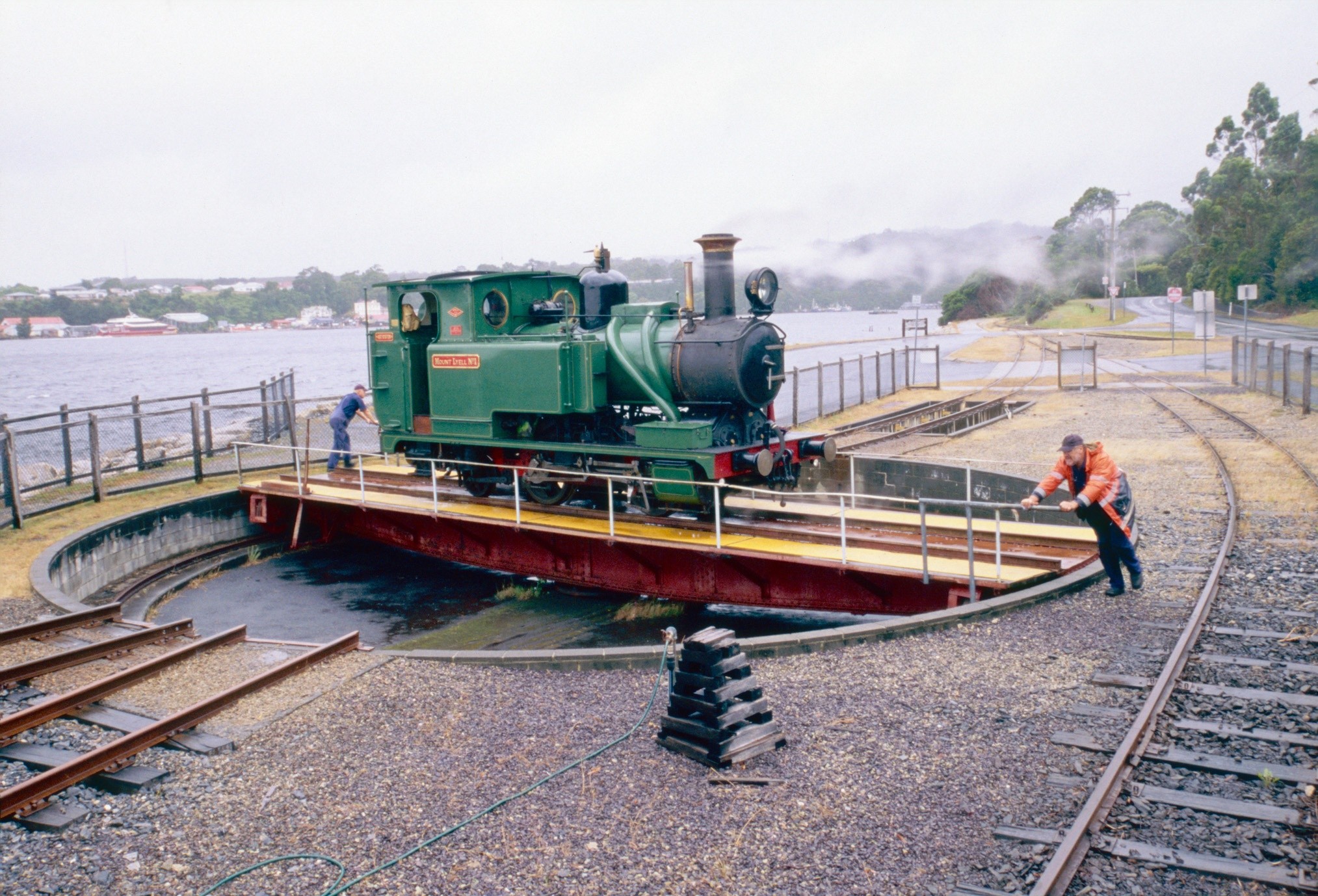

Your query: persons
(1020, 434), (1143, 597)
(326, 384), (379, 473)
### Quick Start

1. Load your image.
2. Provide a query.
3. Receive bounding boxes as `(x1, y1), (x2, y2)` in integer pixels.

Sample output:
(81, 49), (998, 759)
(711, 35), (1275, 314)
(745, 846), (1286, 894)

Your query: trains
(359, 231), (838, 525)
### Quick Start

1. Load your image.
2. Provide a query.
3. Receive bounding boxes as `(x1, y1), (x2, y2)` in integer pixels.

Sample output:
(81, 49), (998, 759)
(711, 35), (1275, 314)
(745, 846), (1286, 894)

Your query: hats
(1056, 434), (1083, 451)
(354, 384), (368, 391)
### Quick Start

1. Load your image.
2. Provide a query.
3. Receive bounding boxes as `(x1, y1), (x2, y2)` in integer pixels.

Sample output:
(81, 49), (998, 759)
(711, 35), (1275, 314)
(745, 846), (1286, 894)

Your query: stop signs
(1167, 287), (1183, 304)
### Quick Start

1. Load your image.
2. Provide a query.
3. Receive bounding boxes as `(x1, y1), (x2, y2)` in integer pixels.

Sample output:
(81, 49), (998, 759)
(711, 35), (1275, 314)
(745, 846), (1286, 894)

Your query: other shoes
(327, 468), (334, 472)
(345, 463), (354, 467)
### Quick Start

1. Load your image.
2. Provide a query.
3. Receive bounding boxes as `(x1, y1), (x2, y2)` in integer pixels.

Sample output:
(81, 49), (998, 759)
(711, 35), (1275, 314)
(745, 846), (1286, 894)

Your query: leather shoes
(1131, 568), (1143, 589)
(1104, 586), (1125, 595)
